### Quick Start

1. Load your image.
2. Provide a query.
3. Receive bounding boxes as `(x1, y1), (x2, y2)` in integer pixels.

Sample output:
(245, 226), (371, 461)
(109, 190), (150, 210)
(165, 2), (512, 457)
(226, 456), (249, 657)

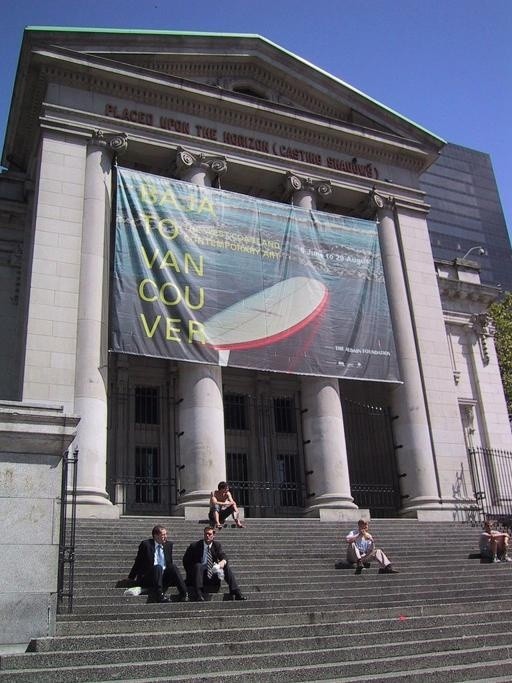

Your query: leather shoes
(193, 593), (205, 601)
(155, 595), (173, 603)
(180, 594), (191, 602)
(234, 594), (247, 600)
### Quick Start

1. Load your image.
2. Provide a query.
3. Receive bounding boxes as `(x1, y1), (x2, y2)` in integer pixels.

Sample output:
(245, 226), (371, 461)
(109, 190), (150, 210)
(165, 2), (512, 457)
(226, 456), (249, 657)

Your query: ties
(156, 544), (164, 574)
(207, 546), (213, 579)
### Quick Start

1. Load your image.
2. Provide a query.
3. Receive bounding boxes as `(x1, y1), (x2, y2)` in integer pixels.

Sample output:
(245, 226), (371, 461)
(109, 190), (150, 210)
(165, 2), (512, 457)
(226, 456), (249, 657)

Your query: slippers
(216, 525), (223, 528)
(237, 525), (246, 528)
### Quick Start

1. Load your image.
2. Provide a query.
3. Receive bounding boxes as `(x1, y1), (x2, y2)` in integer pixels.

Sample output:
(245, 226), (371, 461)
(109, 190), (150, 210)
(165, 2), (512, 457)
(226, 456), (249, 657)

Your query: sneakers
(379, 564), (400, 574)
(491, 551), (512, 563)
(357, 559), (365, 569)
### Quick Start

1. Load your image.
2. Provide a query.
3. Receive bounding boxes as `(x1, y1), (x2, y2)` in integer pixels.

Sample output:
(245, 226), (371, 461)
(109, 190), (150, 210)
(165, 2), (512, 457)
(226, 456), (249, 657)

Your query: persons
(207, 480), (244, 529)
(127, 526), (189, 602)
(345, 519), (394, 573)
(183, 526), (247, 601)
(479, 520), (512, 563)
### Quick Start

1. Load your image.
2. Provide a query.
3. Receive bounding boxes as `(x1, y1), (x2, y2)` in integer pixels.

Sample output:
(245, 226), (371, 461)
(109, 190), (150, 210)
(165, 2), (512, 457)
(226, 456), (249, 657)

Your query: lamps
(463, 246), (488, 261)
